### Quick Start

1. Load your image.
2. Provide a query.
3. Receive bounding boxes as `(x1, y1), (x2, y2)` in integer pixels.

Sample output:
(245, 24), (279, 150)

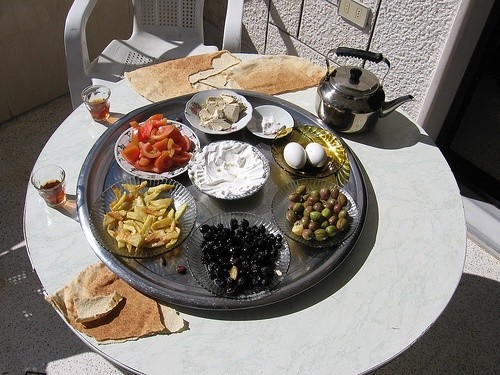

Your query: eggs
(305, 143), (327, 167)
(283, 142), (306, 169)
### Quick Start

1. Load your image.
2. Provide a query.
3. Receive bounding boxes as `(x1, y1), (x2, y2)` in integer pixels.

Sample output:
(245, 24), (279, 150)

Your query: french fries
(102, 181), (189, 252)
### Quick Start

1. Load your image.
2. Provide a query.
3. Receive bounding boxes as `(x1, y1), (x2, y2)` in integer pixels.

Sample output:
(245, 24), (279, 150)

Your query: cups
(30, 163), (67, 208)
(81, 85), (111, 123)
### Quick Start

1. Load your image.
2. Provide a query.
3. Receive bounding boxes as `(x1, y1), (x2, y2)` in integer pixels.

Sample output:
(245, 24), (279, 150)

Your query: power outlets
(338, 0), (370, 29)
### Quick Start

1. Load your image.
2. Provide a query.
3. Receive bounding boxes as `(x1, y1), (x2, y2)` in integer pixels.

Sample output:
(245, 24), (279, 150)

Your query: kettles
(314, 47), (417, 136)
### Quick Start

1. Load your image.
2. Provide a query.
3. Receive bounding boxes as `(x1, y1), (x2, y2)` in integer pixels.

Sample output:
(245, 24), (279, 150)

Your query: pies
(124, 50), (332, 104)
(43, 262), (186, 344)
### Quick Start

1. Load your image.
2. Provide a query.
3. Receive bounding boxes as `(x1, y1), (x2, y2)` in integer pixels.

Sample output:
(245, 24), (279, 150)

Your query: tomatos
(122, 114), (193, 174)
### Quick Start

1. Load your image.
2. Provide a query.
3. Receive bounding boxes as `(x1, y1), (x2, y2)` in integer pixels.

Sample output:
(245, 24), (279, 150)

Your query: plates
(246, 104), (294, 140)
(113, 120), (201, 181)
(270, 177), (361, 249)
(88, 175), (198, 260)
(185, 89), (253, 135)
(187, 140), (271, 200)
(271, 124), (347, 178)
(187, 211), (292, 302)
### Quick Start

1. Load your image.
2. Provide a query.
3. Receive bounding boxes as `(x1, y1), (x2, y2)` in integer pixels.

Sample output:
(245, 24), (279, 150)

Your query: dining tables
(23, 52), (467, 375)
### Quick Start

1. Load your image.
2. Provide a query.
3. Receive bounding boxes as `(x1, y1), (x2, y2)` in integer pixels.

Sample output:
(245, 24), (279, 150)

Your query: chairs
(64, 0), (244, 109)
